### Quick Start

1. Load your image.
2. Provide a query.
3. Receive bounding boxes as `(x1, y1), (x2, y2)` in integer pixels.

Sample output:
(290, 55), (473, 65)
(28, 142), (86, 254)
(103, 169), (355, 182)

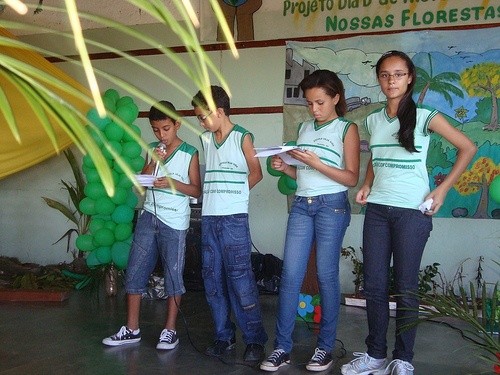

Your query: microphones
(153, 143), (166, 176)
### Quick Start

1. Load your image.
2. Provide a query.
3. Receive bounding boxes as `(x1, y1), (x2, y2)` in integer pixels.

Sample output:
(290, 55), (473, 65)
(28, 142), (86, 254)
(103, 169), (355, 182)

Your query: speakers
(157, 218), (204, 291)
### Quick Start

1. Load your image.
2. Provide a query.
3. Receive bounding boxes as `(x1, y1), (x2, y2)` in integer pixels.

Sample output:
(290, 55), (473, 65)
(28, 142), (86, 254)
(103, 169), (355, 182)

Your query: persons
(190, 86), (269, 366)
(102, 101), (201, 350)
(259, 70), (360, 371)
(341, 50), (477, 375)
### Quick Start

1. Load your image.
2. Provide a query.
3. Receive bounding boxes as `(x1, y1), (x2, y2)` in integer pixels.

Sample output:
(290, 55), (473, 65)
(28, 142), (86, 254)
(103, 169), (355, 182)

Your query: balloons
(266, 141), (298, 195)
(489, 175), (500, 203)
(75, 89), (145, 270)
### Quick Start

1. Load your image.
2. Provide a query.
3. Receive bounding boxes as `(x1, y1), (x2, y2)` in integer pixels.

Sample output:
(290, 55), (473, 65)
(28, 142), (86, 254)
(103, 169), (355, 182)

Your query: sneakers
(259, 349), (290, 371)
(102, 325), (141, 346)
(384, 359), (414, 375)
(243, 343), (267, 361)
(306, 347), (332, 371)
(156, 329), (179, 350)
(202, 338), (236, 356)
(339, 352), (390, 375)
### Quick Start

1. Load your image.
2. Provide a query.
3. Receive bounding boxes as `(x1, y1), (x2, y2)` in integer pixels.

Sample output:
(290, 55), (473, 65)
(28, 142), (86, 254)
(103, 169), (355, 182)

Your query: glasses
(378, 72), (409, 81)
(196, 111), (213, 122)
